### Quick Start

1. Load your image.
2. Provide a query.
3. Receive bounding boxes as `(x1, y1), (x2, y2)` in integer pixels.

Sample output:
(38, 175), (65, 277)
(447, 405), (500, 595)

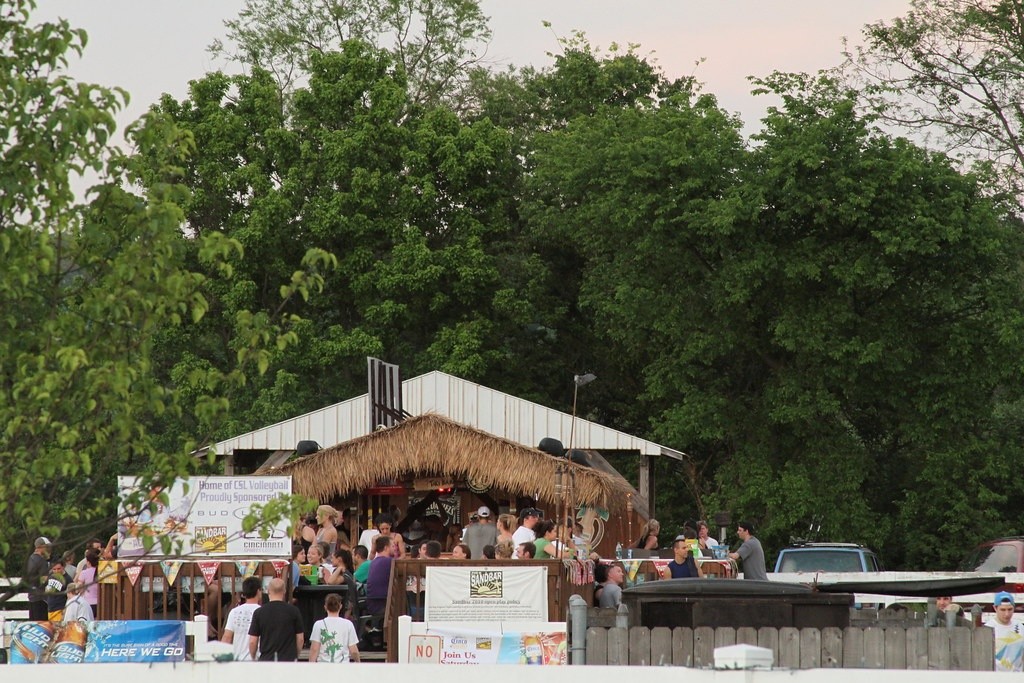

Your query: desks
(293, 585), (350, 648)
(619, 557), (736, 588)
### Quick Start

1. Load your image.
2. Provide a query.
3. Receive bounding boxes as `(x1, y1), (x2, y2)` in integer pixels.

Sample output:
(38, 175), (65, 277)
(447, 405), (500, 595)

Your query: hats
(994, 591), (1014, 607)
(34, 537), (51, 548)
(738, 521), (752, 529)
(64, 583), (76, 593)
(520, 508), (542, 517)
(478, 506), (490, 517)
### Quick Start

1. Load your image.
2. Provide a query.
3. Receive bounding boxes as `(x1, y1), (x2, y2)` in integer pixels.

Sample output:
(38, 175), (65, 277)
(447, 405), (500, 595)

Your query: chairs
(344, 574), (387, 653)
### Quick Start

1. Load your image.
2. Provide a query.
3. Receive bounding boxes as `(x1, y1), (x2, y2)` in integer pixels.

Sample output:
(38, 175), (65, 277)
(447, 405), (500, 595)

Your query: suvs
(775, 542), (885, 573)
(959, 537), (1024, 613)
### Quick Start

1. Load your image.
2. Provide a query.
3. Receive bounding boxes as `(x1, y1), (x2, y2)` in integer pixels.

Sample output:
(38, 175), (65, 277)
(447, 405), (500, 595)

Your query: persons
(594, 566), (608, 606)
(664, 539), (703, 578)
(221, 577), (262, 661)
(289, 506), (587, 633)
(599, 565), (624, 609)
(308, 593), (360, 662)
(984, 591), (1024, 671)
(626, 519), (661, 550)
(937, 596), (952, 610)
(76, 533), (118, 573)
(728, 523), (769, 580)
(62, 551), (77, 579)
(45, 561), (74, 621)
(28, 537), (52, 621)
(248, 578), (305, 661)
(78, 548), (98, 618)
(64, 583), (94, 620)
(674, 521), (718, 549)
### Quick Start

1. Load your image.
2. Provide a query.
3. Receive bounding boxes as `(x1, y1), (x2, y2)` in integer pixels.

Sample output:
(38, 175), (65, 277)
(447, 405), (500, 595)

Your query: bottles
(627, 539), (633, 558)
(615, 542), (622, 560)
